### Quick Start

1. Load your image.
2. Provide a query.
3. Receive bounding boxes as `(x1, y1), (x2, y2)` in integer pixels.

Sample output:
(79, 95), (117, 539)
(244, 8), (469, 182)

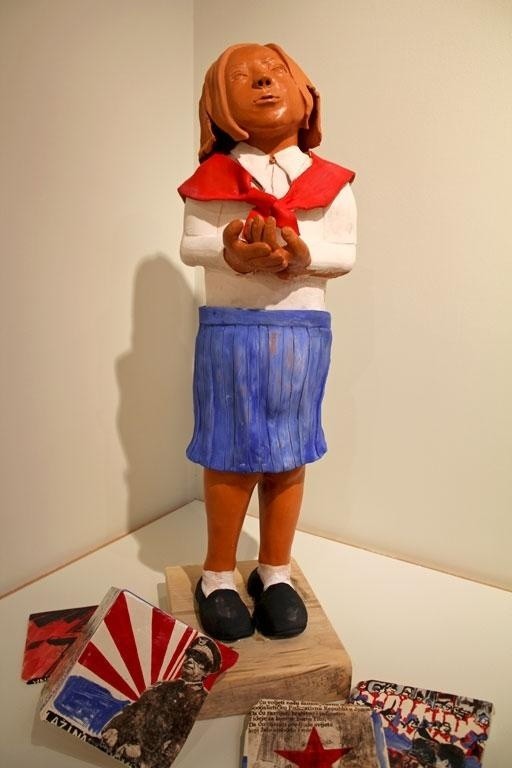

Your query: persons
(104, 634), (226, 768)
(358, 676), (501, 768)
(175, 38), (363, 646)
